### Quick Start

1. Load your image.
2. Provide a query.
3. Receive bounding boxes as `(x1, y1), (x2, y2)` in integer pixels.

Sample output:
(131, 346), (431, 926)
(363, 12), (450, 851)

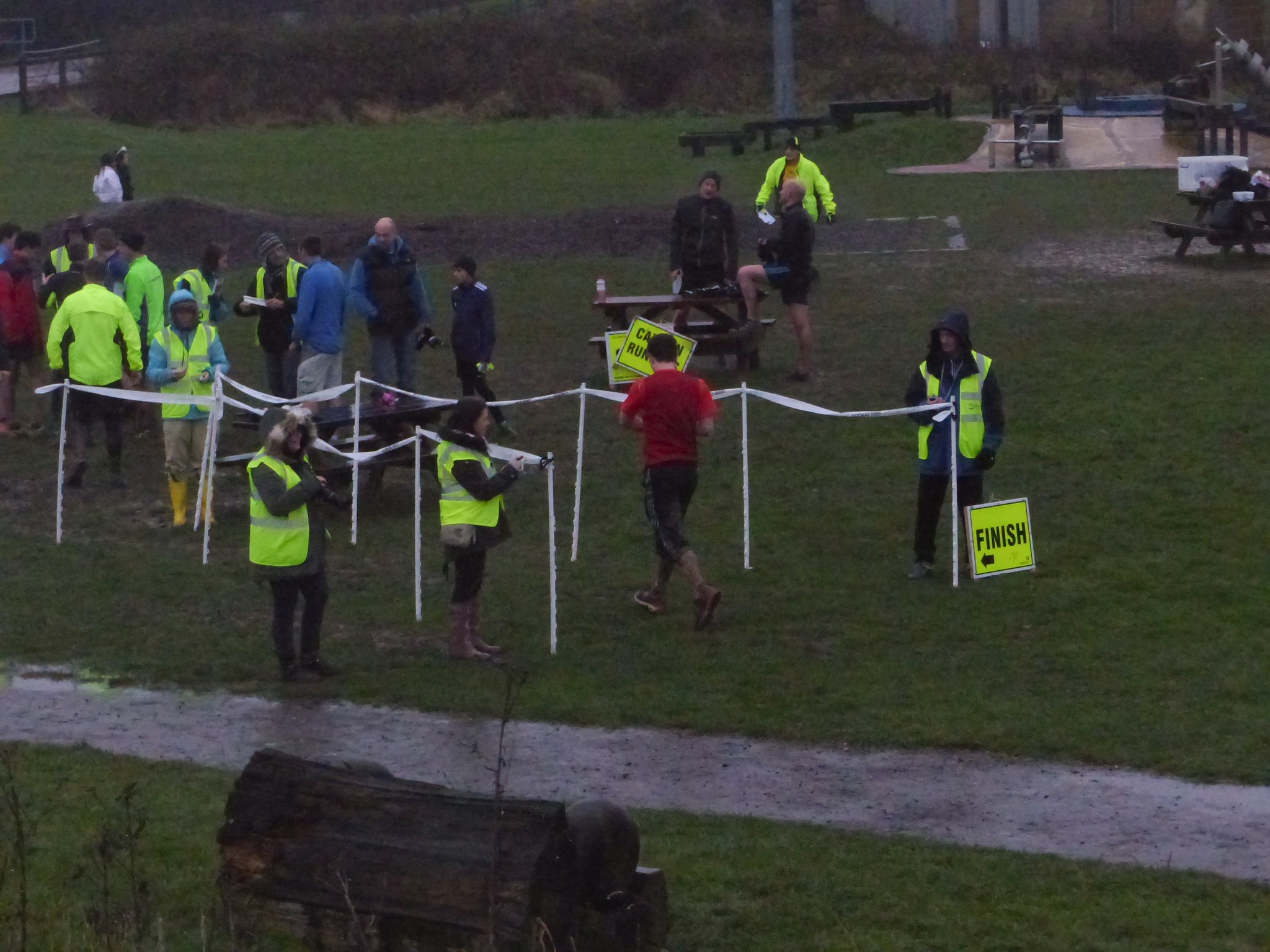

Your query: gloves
(757, 204), (767, 217)
(974, 445), (997, 471)
(826, 213), (835, 226)
(509, 453), (528, 472)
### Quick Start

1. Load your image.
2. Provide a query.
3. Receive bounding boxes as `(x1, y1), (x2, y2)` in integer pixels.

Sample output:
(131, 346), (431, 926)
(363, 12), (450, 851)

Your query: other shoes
(728, 319), (766, 339)
(493, 426), (516, 445)
(299, 658), (343, 675)
(783, 368), (811, 382)
(281, 666), (322, 683)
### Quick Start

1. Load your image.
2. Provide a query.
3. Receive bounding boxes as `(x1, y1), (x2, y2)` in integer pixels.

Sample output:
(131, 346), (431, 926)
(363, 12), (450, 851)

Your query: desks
(232, 391), (457, 493)
(593, 290), (771, 368)
(1174, 191), (1270, 259)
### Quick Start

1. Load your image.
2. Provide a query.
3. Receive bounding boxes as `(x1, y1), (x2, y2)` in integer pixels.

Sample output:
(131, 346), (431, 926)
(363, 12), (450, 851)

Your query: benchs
(589, 317), (777, 346)
(1149, 218), (1234, 242)
(315, 448), (440, 478)
(215, 433), (383, 470)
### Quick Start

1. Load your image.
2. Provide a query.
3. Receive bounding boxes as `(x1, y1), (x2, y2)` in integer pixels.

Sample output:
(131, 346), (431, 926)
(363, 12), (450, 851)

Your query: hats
(699, 171), (721, 192)
(256, 231), (290, 267)
(787, 137), (801, 150)
(454, 257), (477, 277)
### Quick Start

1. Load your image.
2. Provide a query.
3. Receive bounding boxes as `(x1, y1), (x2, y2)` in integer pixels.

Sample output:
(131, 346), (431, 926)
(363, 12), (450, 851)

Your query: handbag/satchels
(440, 523), (477, 548)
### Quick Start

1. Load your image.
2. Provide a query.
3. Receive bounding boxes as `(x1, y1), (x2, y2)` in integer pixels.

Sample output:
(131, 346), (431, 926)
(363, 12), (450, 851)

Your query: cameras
(315, 481), (350, 512)
(416, 326), (436, 350)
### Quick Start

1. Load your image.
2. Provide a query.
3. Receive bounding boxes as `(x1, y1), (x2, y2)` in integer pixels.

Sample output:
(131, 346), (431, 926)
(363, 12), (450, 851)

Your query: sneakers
(634, 585), (665, 614)
(960, 561), (971, 574)
(691, 584), (722, 630)
(906, 561), (934, 579)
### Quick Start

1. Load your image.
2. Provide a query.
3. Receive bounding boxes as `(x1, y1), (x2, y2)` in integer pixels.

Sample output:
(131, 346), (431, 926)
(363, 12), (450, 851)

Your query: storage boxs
(1177, 155), (1248, 192)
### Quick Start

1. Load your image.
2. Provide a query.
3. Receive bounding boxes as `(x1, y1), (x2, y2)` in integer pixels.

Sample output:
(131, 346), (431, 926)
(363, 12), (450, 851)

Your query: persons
(904, 315), (1007, 578)
(615, 334), (720, 634)
(242, 407), (344, 680)
(437, 398), (523, 660)
(1, 132), (841, 533)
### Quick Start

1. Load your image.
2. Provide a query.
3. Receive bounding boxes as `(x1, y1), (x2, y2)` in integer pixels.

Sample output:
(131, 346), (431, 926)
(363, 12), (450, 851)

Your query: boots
(199, 478), (217, 525)
(447, 602), (491, 661)
(168, 472), (188, 528)
(469, 594), (502, 654)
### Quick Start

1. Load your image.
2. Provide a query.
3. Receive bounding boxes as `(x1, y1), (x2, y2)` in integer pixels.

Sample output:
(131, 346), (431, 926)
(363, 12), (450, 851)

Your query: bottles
(1200, 175), (1206, 186)
(596, 275), (606, 304)
